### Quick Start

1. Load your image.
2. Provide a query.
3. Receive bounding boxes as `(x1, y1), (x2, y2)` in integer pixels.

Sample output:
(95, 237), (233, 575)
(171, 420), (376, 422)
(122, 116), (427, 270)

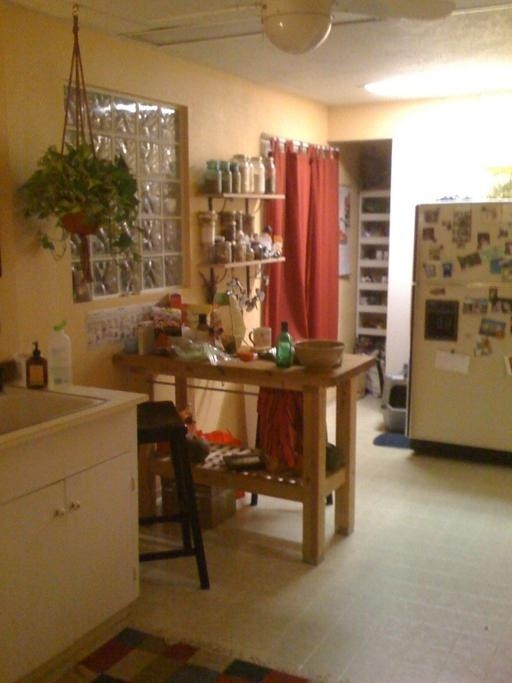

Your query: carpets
(50, 628), (314, 683)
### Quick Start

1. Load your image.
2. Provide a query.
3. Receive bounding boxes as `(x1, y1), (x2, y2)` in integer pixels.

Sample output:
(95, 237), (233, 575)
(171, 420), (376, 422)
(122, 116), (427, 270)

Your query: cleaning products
(25, 341), (47, 387)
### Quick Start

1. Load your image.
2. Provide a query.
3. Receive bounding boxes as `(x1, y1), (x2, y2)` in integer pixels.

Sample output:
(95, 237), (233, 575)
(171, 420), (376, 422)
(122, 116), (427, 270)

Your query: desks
(113, 353), (378, 567)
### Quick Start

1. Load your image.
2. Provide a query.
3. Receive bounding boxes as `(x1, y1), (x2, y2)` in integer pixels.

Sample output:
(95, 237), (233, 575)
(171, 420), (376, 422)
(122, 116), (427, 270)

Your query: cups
(249, 327), (272, 350)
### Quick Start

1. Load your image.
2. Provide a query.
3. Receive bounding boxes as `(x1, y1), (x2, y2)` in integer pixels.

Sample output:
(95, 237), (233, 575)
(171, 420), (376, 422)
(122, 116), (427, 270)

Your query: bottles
(276, 322), (292, 369)
(204, 153), (277, 194)
(197, 313), (209, 343)
(198, 209), (272, 264)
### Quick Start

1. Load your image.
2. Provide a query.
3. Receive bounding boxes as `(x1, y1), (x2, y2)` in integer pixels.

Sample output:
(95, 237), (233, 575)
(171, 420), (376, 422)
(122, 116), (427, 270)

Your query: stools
(137, 401), (210, 592)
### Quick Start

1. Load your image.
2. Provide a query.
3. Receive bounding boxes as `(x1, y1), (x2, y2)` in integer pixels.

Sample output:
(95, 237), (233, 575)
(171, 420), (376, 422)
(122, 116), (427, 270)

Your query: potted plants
(16, 142), (151, 273)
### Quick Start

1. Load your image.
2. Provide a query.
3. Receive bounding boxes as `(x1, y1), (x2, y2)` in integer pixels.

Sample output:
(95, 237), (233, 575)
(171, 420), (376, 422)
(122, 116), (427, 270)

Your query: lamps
(258, 0), (336, 56)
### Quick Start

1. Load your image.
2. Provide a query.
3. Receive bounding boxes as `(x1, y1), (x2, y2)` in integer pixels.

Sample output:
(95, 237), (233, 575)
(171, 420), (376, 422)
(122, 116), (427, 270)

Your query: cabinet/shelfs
(198, 189), (286, 269)
(355, 188), (391, 355)
(1, 407), (139, 683)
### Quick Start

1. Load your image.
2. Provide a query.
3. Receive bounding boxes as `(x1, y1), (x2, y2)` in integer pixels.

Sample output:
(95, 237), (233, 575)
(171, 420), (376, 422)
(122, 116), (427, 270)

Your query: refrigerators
(404, 203), (512, 456)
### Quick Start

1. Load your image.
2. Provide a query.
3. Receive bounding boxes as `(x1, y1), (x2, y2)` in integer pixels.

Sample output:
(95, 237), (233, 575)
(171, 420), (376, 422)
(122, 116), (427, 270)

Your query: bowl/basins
(294, 340), (344, 369)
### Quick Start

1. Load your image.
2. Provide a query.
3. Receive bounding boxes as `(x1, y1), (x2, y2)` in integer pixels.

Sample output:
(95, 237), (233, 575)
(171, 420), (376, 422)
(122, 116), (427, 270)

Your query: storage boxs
(161, 476), (237, 529)
